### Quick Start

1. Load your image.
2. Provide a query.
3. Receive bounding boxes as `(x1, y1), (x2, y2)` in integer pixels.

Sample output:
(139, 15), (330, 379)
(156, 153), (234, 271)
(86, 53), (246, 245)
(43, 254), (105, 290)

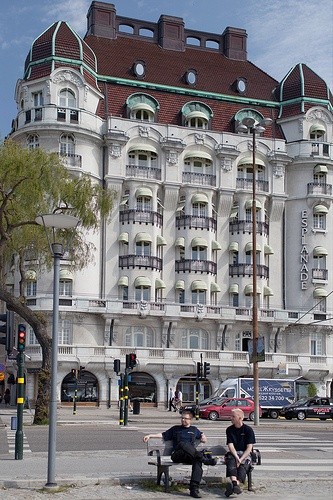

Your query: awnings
(5, 268), (72, 282)
(310, 124), (327, 297)
(114, 103), (274, 296)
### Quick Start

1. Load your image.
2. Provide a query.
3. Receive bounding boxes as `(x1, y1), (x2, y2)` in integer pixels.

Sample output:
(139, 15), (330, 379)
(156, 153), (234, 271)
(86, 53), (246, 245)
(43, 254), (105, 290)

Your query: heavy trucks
(198, 378), (297, 418)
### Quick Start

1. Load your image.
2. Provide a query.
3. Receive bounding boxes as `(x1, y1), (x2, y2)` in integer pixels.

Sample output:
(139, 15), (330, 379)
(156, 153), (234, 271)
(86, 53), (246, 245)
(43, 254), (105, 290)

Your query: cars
(282, 397), (333, 421)
(199, 397), (263, 421)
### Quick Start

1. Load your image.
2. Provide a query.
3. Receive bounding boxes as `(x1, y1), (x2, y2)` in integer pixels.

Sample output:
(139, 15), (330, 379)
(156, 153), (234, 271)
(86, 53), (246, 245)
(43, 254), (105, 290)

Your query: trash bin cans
(132, 398), (141, 414)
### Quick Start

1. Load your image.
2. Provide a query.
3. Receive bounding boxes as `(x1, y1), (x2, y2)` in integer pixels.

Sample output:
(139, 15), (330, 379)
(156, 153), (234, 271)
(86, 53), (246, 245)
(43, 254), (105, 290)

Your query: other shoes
(224, 482), (234, 498)
(190, 490), (202, 498)
(173, 407), (178, 412)
(204, 457), (217, 466)
(233, 485), (243, 495)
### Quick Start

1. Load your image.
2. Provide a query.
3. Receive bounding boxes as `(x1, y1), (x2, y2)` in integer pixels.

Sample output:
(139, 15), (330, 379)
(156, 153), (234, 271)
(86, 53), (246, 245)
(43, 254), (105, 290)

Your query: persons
(120, 389), (136, 414)
(221, 408), (255, 497)
(4, 389), (10, 407)
(143, 411), (219, 498)
(167, 388), (185, 412)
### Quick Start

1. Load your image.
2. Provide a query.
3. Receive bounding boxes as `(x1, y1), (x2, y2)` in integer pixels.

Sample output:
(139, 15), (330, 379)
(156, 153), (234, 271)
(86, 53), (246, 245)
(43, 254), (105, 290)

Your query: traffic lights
(18, 324), (26, 352)
(129, 353), (137, 367)
(0, 371), (5, 381)
(204, 362), (211, 378)
(197, 362), (203, 377)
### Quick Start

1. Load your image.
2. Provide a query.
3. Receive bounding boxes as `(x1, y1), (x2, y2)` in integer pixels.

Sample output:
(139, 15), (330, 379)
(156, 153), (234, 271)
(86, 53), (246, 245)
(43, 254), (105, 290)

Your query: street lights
(34, 213), (80, 488)
(240, 117), (266, 426)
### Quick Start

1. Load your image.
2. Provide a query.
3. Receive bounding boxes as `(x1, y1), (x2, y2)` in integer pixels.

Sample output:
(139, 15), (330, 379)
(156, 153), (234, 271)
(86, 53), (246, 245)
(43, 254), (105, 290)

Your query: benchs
(147, 436), (262, 492)
